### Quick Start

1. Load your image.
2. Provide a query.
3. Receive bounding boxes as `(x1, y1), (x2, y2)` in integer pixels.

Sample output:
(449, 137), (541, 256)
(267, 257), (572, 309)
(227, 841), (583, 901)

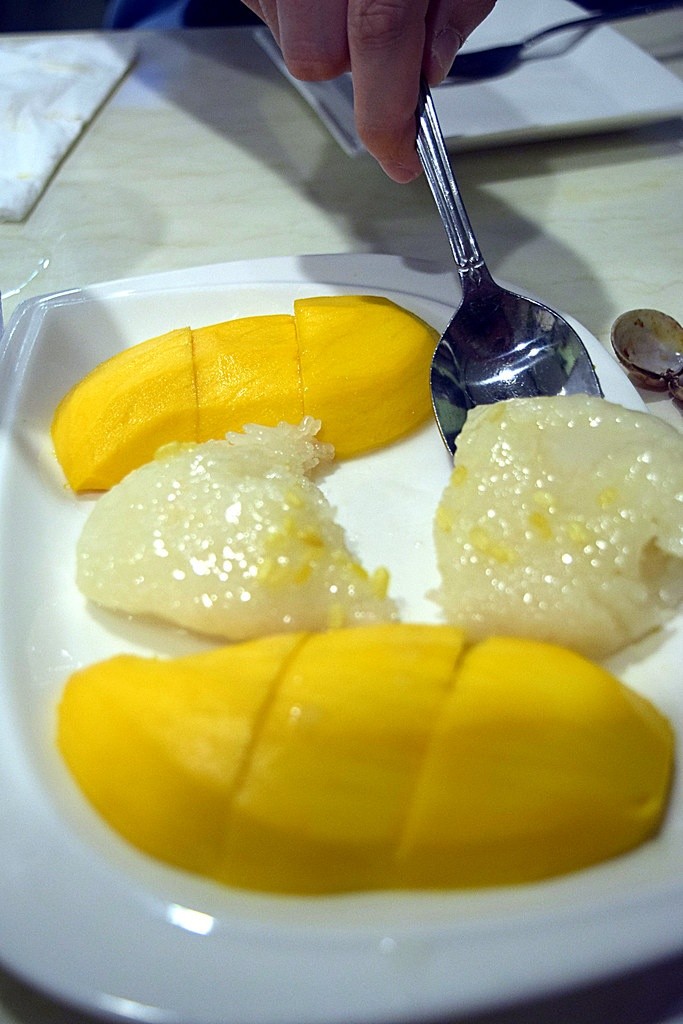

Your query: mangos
(55, 620), (674, 894)
(49, 296), (446, 494)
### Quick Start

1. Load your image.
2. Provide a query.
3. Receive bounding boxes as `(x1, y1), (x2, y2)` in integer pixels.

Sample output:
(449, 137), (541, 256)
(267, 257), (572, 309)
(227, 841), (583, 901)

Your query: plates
(0, 253), (683, 1024)
(257, 0), (683, 159)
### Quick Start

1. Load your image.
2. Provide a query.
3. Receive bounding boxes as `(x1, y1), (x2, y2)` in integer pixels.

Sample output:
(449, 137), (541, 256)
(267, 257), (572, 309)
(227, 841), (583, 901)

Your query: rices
(73, 418), (402, 638)
(425, 393), (683, 658)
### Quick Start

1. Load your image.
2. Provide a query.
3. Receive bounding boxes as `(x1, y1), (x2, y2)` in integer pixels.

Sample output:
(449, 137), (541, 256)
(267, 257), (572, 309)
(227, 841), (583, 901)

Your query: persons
(240, 1), (497, 182)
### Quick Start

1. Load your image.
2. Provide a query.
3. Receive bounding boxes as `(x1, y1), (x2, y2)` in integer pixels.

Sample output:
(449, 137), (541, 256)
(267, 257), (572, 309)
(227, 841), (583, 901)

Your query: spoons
(414, 73), (606, 463)
(436, 0), (683, 86)
